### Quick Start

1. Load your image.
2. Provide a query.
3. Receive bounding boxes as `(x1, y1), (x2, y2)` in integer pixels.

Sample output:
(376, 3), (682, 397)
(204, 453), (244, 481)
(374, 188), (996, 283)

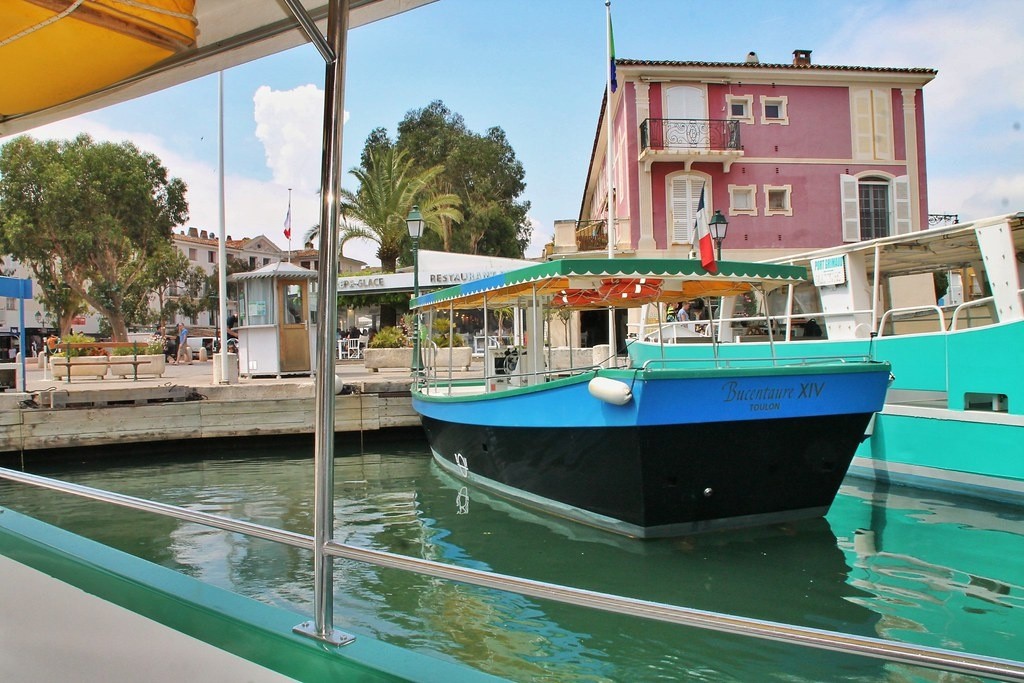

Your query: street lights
(405, 205), (426, 377)
(708, 210), (730, 317)
(35, 311), (52, 350)
(209, 292), (219, 353)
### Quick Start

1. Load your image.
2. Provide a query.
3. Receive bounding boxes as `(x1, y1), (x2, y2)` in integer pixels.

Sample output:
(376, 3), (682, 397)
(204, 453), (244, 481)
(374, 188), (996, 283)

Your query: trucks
(186, 337), (220, 360)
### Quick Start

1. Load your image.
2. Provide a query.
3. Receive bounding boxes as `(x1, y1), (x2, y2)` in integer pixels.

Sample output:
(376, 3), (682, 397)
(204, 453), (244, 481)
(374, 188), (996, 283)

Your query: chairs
(746, 312), (764, 335)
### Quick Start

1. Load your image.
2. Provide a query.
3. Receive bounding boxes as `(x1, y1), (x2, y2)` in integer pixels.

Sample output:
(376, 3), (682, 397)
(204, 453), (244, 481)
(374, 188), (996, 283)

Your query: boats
(410, 258), (891, 542)
(625, 212), (1023, 506)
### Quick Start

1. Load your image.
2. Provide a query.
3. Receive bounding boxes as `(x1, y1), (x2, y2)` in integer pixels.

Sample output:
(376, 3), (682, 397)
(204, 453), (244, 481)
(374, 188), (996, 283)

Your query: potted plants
(363, 314), (472, 373)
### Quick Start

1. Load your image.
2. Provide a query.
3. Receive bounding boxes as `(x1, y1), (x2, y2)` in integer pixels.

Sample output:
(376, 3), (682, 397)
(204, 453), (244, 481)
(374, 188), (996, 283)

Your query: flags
(692, 185), (718, 274)
(610, 13), (617, 93)
(283, 206), (291, 240)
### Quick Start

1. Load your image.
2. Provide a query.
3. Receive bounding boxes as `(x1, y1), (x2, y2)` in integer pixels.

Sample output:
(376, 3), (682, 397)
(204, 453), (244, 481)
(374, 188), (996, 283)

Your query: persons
(335, 326), (376, 359)
(154, 312), (238, 366)
(32, 332), (60, 359)
(667, 300), (691, 323)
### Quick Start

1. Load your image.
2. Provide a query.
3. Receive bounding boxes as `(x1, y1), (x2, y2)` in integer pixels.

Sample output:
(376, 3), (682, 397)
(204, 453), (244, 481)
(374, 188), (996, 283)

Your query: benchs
(48, 341), (165, 380)
